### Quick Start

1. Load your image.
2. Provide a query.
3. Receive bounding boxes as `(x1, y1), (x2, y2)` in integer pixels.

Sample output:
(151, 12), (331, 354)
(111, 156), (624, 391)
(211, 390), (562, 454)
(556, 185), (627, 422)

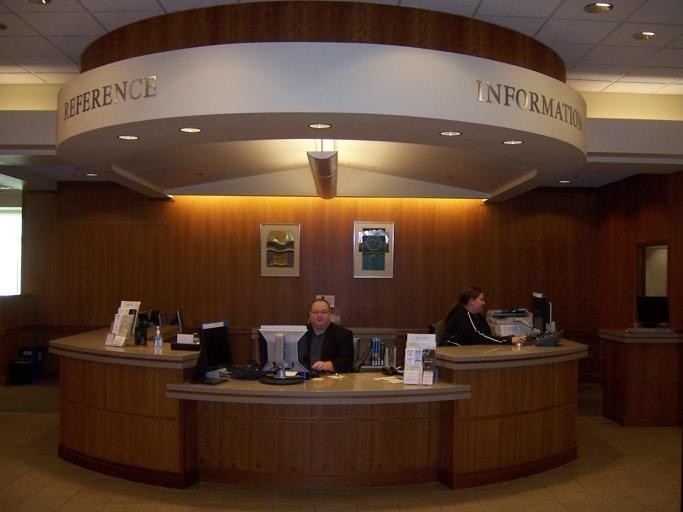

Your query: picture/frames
(257, 220), (302, 279)
(351, 220), (396, 282)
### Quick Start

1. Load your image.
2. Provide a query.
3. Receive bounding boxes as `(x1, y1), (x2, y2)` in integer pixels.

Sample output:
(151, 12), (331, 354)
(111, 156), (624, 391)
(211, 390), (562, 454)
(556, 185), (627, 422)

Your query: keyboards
(628, 328), (675, 333)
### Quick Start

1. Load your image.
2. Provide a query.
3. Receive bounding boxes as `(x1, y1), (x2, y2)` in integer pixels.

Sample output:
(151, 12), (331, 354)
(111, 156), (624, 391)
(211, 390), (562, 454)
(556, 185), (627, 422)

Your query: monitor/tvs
(532, 292), (552, 338)
(636, 296), (670, 328)
(138, 309), (183, 329)
(258, 325), (311, 385)
(190, 321), (232, 385)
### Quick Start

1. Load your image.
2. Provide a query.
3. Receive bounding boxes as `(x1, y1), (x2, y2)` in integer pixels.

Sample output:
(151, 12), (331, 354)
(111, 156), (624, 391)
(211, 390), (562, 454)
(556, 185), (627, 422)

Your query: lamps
(304, 137), (340, 202)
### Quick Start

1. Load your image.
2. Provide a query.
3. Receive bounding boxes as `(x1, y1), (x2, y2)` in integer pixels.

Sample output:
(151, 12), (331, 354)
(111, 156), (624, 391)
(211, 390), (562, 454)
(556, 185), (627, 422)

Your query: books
(370, 336), (384, 366)
(403, 333), (437, 385)
(111, 300), (142, 340)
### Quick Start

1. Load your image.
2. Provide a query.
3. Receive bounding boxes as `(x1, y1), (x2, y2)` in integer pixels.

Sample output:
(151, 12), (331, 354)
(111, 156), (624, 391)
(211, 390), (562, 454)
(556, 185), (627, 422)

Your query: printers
(486, 308), (533, 337)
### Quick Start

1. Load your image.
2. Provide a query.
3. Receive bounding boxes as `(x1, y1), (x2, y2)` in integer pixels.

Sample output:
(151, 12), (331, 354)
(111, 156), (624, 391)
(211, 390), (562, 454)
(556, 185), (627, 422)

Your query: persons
(439, 286), (527, 347)
(297, 298), (355, 374)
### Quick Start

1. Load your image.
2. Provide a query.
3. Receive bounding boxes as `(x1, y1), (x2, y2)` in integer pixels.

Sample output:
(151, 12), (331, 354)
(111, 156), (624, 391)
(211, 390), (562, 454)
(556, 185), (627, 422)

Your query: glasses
(310, 310), (330, 315)
